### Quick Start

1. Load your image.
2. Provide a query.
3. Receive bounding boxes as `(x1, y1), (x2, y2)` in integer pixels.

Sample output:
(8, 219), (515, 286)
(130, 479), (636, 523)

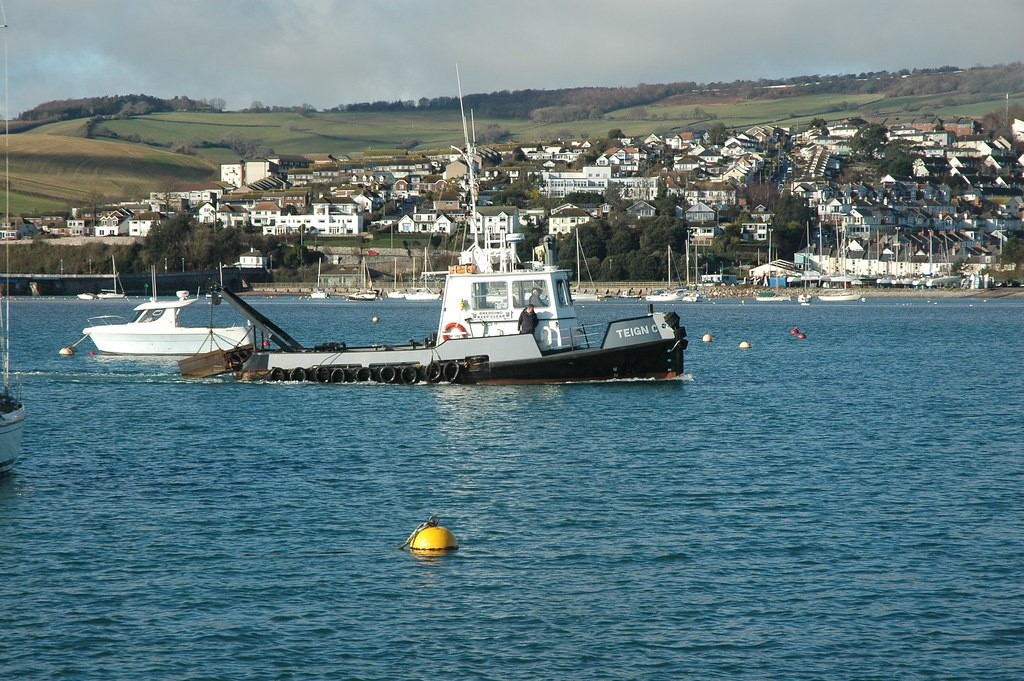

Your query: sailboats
(555, 227), (598, 302)
(755, 228), (793, 302)
(309, 257), (330, 299)
(797, 221), (811, 303)
(646, 245), (684, 303)
(682, 229), (700, 302)
(817, 221), (863, 301)
(876, 230), (963, 288)
(97, 254), (125, 299)
(348, 247), (441, 302)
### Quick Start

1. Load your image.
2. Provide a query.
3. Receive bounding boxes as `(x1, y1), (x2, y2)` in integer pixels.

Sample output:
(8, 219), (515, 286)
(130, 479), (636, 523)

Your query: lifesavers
(401, 367), (418, 385)
(355, 367), (372, 383)
(290, 366), (308, 382)
(443, 361), (460, 382)
(331, 368), (346, 383)
(270, 367), (286, 382)
(315, 366), (330, 383)
(443, 322), (465, 340)
(425, 362), (442, 382)
(380, 366), (396, 382)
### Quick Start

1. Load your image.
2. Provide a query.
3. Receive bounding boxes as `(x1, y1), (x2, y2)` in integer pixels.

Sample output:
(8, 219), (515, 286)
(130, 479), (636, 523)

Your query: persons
(517, 304), (539, 334)
(529, 288), (547, 306)
(762, 271), (766, 283)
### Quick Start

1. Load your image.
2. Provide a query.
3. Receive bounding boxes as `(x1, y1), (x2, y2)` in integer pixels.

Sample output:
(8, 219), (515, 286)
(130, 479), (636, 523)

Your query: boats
(78, 292), (99, 301)
(618, 291), (641, 298)
(82, 289), (252, 355)
(822, 282), (830, 289)
(850, 279), (863, 288)
(0, 386), (25, 474)
(220, 63), (686, 386)
